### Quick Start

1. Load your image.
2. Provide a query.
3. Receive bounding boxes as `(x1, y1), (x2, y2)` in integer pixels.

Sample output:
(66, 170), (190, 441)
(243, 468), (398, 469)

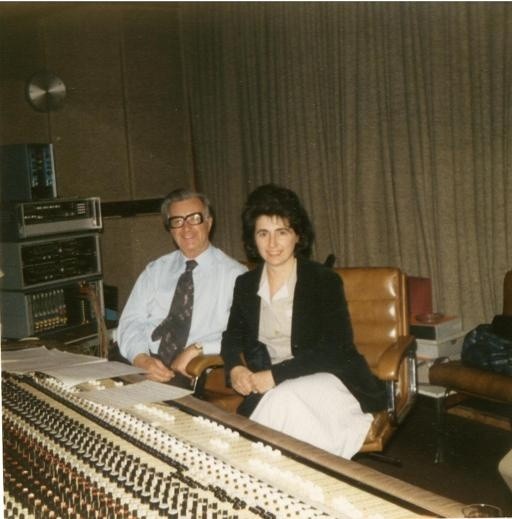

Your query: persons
(221, 184), (392, 461)
(115, 188), (250, 391)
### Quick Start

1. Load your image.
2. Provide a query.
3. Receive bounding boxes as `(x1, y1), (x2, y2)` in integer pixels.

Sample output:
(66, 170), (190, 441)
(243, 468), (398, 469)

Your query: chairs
(430, 268), (512, 436)
(187, 264), (410, 467)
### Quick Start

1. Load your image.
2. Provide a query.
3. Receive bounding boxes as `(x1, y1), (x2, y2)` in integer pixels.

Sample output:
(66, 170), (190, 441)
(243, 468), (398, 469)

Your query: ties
(151, 259), (199, 371)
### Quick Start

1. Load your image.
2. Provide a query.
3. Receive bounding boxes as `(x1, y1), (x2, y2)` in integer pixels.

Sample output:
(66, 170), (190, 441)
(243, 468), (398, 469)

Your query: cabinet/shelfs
(414, 332), (471, 429)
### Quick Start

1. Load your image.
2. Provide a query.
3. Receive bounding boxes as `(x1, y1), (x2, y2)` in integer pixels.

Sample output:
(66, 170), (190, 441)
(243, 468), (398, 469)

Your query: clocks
(25, 72), (66, 112)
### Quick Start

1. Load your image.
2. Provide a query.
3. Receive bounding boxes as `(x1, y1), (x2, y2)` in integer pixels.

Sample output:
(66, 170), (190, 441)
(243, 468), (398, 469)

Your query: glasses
(167, 212), (206, 229)
